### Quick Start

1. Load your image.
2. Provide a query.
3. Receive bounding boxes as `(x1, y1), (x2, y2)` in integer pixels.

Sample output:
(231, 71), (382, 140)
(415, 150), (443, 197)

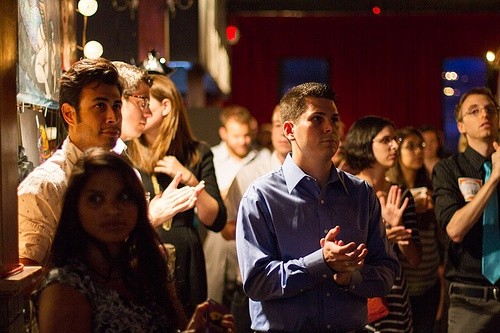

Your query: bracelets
(334, 275), (352, 289)
(180, 172), (192, 184)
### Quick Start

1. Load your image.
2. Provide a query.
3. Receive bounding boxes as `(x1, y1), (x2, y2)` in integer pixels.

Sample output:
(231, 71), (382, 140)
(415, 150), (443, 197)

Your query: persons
(18, 57), (500, 333)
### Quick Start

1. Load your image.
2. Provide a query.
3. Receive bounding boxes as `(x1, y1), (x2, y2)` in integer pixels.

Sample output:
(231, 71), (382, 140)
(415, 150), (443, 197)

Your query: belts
(451, 286), (500, 303)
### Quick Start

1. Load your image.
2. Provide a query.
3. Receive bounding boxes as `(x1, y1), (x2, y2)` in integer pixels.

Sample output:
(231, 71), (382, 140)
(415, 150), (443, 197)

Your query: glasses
(128, 93), (150, 111)
(406, 141), (426, 149)
(374, 135), (402, 145)
(458, 105), (497, 122)
